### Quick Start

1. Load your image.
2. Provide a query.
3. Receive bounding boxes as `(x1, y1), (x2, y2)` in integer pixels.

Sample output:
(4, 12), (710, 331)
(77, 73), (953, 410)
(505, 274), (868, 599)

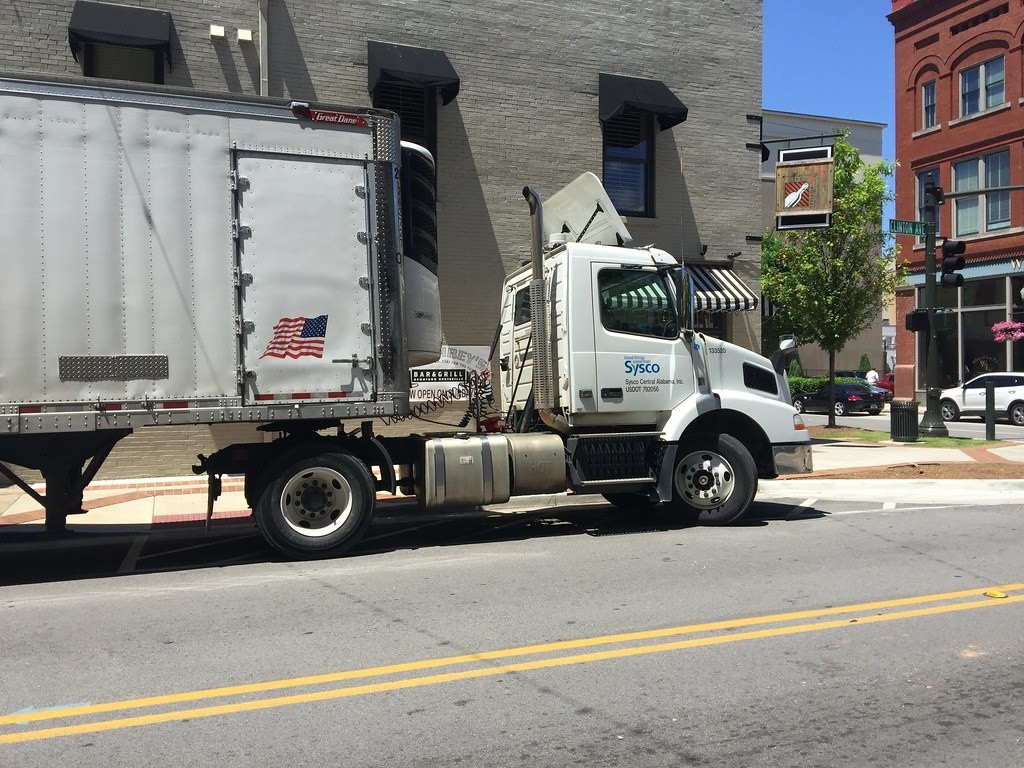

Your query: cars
(834, 371), (867, 380)
(854, 378), (894, 402)
(792, 384), (884, 417)
(876, 373), (894, 392)
(939, 372), (1024, 426)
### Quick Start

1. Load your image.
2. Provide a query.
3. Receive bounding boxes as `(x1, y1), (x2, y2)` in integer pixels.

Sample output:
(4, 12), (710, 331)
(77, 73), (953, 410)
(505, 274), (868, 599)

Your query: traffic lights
(906, 311), (928, 332)
(941, 240), (966, 288)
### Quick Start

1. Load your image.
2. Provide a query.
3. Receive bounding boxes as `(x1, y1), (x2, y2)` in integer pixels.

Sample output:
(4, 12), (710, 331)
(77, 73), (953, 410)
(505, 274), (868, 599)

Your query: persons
(866, 367), (880, 387)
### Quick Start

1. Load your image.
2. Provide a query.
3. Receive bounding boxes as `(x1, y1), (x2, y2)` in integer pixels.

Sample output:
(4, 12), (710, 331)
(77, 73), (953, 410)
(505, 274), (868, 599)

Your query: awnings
(599, 73), (688, 131)
(762, 143), (771, 161)
(67, 1), (171, 62)
(604, 266), (759, 311)
(367, 40), (460, 107)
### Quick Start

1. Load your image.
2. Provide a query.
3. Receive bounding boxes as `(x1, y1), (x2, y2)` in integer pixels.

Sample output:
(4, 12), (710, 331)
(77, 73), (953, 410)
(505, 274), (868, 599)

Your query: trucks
(0, 68), (813, 560)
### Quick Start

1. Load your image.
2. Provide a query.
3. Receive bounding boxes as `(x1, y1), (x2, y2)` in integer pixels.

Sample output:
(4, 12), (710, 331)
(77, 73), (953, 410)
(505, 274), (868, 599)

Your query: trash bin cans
(885, 401), (922, 442)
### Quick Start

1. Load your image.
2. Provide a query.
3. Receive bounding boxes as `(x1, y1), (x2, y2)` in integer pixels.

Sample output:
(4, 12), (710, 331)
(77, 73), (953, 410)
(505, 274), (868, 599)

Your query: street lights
(882, 338), (887, 374)
